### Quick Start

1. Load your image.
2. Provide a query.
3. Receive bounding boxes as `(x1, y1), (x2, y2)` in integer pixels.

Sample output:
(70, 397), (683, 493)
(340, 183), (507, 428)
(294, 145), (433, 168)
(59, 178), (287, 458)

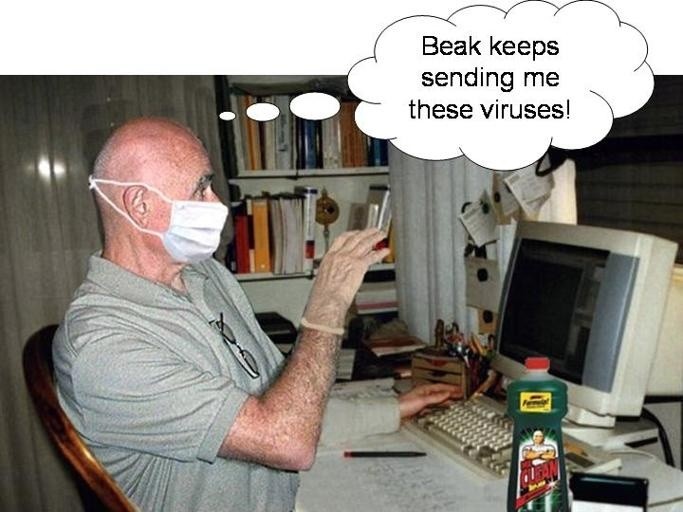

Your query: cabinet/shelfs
(213, 75), (399, 312)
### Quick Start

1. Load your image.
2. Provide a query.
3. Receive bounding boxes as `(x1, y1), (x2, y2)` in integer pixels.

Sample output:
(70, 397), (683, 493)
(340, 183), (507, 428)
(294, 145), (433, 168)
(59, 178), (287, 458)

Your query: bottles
(503, 355), (572, 512)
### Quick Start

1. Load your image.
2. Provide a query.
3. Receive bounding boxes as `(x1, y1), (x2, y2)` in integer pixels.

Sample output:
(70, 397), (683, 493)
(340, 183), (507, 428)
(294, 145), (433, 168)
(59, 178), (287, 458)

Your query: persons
(51, 115), (466, 512)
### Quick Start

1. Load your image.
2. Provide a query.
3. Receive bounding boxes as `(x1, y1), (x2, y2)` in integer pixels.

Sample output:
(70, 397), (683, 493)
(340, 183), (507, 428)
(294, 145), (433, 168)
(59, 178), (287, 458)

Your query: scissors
(449, 342), (473, 368)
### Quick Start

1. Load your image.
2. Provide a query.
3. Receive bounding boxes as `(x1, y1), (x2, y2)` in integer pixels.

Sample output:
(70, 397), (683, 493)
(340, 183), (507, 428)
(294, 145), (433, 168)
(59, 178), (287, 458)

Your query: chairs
(22, 325), (137, 512)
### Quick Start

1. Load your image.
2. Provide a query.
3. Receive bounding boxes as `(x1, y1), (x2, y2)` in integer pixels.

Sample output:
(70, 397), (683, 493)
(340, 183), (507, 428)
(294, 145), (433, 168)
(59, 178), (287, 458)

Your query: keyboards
(399, 396), (622, 486)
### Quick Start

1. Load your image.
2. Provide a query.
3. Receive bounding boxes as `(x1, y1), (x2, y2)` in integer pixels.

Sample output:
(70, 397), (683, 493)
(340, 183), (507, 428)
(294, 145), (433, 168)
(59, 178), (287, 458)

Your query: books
(229, 92), (427, 381)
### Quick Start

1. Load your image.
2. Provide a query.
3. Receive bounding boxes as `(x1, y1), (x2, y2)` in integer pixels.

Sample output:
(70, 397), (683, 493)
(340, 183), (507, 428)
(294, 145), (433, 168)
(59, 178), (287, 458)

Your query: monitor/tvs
(487, 218), (683, 447)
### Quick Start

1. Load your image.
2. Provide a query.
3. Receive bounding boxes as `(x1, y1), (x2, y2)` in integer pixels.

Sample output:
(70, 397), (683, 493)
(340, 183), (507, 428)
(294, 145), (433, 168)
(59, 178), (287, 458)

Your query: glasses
(210, 313), (262, 378)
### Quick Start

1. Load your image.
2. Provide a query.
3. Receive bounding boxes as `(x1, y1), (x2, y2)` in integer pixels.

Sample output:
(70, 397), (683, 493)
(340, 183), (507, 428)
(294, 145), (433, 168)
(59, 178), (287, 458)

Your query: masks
(160, 200), (228, 264)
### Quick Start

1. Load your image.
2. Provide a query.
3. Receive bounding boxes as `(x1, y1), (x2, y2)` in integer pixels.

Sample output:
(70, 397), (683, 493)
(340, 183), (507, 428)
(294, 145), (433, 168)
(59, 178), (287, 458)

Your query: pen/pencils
(344, 452), (428, 458)
